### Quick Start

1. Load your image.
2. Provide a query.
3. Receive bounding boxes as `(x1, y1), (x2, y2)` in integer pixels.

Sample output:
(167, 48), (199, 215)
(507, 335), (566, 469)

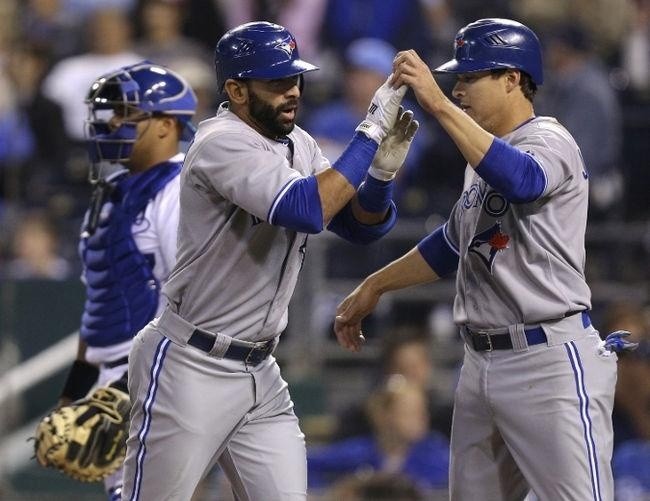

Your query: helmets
(82, 60), (197, 188)
(430, 18), (543, 85)
(214, 21), (319, 97)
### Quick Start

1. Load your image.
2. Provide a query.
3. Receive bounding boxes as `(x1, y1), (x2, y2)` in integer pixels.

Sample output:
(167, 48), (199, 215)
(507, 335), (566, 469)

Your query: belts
(187, 329), (275, 367)
(458, 310), (592, 353)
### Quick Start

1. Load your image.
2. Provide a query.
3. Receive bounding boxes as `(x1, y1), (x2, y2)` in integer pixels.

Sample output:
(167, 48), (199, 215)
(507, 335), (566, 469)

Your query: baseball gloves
(34, 387), (131, 483)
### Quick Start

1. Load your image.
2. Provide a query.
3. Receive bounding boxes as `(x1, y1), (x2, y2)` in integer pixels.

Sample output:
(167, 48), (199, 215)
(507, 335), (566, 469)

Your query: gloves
(599, 330), (640, 357)
(366, 104), (419, 181)
(354, 73), (409, 146)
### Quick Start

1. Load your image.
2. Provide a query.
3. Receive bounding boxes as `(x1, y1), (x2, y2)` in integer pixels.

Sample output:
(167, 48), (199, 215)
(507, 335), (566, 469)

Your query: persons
(1, 0), (650, 282)
(38, 58), (205, 501)
(327, 15), (620, 498)
(122, 19), (420, 500)
(302, 289), (649, 501)
